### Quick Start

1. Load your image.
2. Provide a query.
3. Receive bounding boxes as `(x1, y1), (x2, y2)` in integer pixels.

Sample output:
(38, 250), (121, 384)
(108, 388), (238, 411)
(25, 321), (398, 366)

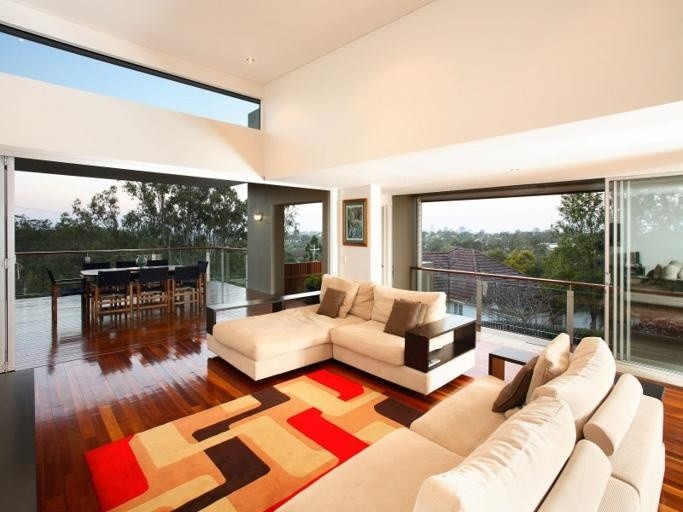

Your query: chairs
(44, 254), (212, 324)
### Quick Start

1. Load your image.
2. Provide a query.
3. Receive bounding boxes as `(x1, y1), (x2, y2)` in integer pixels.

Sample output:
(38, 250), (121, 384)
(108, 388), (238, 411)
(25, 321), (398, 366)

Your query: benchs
(486, 344), (665, 402)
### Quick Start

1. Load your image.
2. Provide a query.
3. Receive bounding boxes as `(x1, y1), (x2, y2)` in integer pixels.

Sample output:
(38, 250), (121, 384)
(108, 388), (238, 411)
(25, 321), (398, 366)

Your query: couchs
(270, 395), (642, 512)
(205, 271), (478, 396)
(409, 330), (666, 512)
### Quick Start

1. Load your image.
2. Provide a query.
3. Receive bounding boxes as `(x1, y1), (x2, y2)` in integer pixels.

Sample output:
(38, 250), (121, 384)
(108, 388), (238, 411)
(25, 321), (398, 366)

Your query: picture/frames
(340, 197), (369, 248)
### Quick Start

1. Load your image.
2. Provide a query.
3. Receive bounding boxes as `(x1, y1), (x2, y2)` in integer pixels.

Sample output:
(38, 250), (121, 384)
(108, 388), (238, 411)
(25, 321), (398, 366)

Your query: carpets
(81, 368), (424, 512)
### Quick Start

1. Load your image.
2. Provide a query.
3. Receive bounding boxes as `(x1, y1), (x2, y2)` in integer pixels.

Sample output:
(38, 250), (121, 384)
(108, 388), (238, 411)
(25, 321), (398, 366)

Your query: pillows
(316, 273), (379, 322)
(526, 332), (572, 405)
(492, 353), (541, 413)
(316, 286), (347, 318)
(381, 299), (422, 336)
(370, 284), (447, 331)
(321, 275), (359, 320)
(413, 302), (430, 328)
